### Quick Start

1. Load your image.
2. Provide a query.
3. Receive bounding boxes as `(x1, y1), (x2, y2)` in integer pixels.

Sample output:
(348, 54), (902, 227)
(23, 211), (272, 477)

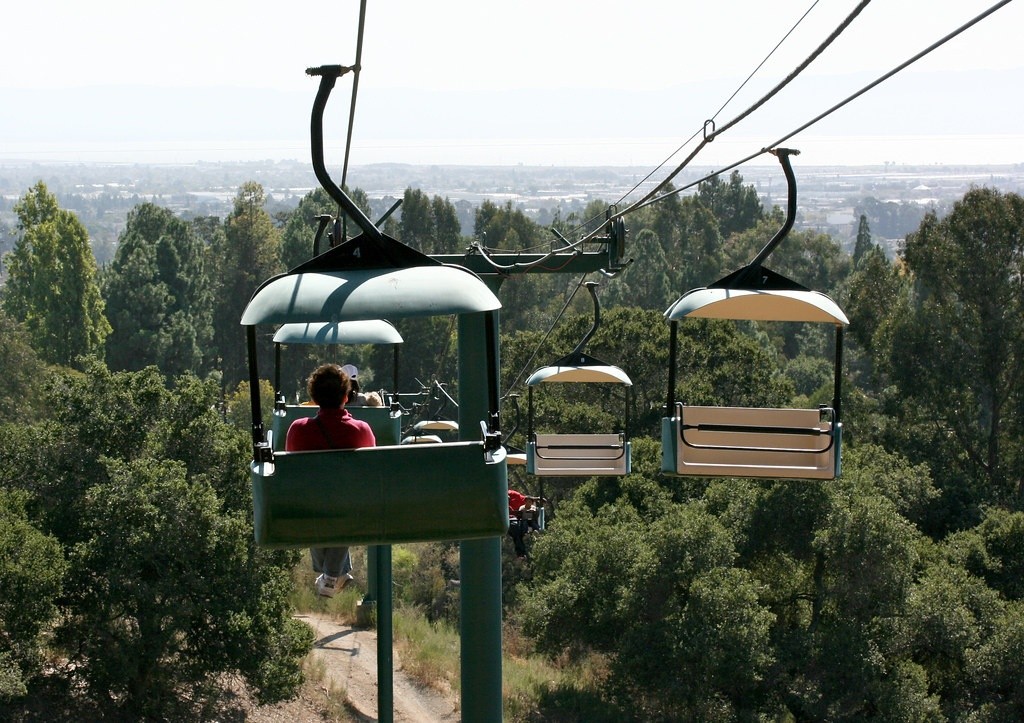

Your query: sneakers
(315, 574), (334, 598)
(336, 573), (353, 594)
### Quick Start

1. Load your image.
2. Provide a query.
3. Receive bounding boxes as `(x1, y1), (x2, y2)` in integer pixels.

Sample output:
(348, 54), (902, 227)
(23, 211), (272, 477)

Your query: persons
(507, 489), (547, 560)
(302, 365), (383, 407)
(286, 363), (376, 599)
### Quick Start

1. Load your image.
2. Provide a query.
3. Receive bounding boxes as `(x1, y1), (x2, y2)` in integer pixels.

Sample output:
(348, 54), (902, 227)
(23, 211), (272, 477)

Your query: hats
(341, 364), (358, 380)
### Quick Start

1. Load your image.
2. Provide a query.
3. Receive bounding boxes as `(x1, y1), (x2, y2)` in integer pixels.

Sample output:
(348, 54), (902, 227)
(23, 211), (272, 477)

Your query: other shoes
(533, 529), (539, 537)
(519, 554), (526, 559)
